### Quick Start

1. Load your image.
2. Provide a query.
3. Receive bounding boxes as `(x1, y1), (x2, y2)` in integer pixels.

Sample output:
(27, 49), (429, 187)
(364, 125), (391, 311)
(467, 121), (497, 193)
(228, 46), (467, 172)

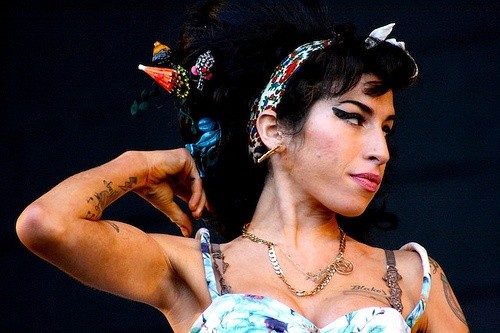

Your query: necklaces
(242, 221), (353, 296)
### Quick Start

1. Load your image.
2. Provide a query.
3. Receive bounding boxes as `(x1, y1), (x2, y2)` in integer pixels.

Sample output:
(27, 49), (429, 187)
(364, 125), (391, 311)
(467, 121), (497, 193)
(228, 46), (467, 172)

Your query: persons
(15, 1), (469, 333)
